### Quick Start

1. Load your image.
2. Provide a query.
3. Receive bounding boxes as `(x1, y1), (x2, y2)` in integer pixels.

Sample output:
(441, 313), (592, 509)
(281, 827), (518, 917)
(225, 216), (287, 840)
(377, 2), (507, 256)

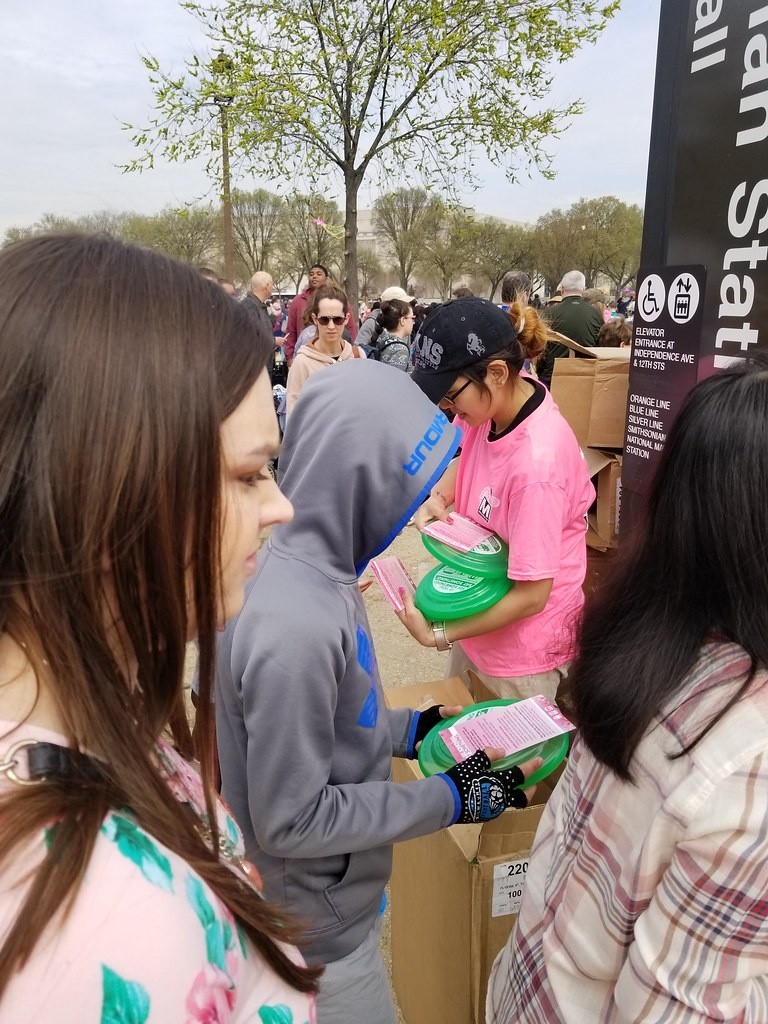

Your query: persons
(192, 265), (635, 1024)
(487, 353), (768, 1024)
(0, 233), (318, 1024)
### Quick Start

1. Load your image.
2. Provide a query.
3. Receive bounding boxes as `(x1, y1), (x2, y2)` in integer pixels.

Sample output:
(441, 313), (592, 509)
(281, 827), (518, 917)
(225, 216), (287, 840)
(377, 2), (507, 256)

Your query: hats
(380, 286), (415, 303)
(410, 296), (518, 404)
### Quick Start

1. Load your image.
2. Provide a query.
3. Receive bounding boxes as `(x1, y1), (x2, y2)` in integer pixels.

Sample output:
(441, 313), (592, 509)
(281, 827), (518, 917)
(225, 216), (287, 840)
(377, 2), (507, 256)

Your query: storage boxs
(549, 327), (631, 597)
(382, 668), (568, 1024)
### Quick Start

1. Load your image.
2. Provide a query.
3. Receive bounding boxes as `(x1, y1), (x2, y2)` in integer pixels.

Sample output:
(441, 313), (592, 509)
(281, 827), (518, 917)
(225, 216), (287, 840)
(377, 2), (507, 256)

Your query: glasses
(405, 315), (416, 321)
(443, 368), (485, 404)
(316, 316), (347, 325)
